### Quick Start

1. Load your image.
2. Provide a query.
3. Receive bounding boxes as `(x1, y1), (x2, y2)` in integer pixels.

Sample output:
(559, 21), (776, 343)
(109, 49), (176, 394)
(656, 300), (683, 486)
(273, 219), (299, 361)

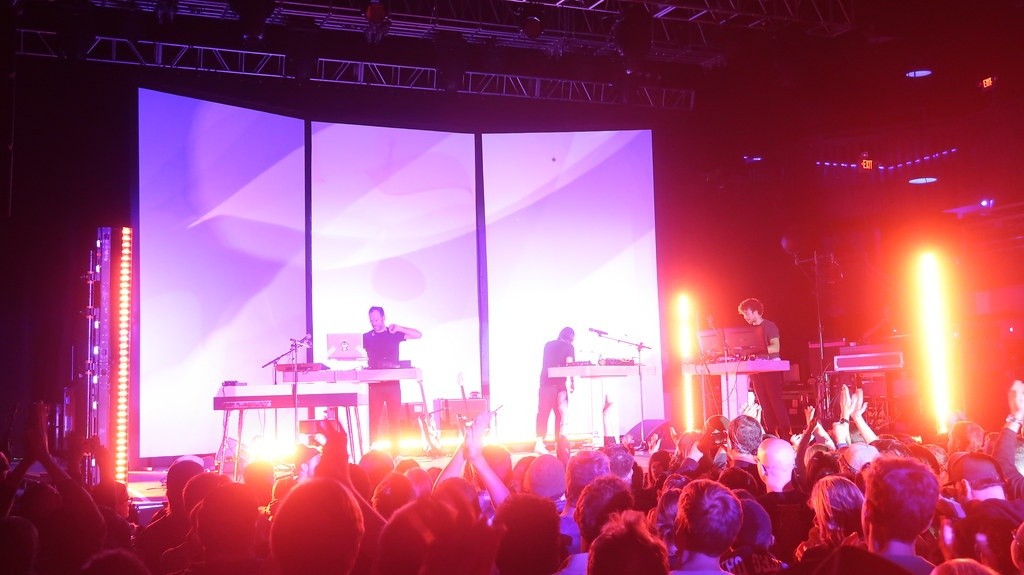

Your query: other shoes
(534, 440), (549, 454)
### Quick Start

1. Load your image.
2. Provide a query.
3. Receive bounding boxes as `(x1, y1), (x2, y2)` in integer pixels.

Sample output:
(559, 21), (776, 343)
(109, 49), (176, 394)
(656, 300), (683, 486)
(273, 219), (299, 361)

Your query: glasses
(122, 497), (134, 503)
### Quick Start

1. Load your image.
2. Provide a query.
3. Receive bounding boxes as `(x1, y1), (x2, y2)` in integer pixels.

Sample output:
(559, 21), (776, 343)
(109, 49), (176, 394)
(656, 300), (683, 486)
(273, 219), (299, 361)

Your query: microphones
(292, 334), (312, 349)
(589, 328), (608, 335)
(290, 339), (310, 349)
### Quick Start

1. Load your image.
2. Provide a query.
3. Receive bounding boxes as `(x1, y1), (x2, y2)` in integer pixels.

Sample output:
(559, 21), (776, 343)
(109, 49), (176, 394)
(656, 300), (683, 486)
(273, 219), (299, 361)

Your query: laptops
(328, 334), (365, 360)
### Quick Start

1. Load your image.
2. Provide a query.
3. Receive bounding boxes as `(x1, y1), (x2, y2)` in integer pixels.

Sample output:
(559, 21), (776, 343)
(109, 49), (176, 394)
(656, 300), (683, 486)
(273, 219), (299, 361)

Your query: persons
(363, 306), (423, 462)
(0, 384), (1023, 575)
(738, 298), (793, 444)
(534, 327), (575, 454)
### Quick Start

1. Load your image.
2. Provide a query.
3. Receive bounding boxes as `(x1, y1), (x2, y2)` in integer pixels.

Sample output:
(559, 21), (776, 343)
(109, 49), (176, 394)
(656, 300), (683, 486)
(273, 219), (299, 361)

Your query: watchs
(839, 418), (850, 423)
(1005, 415), (1022, 425)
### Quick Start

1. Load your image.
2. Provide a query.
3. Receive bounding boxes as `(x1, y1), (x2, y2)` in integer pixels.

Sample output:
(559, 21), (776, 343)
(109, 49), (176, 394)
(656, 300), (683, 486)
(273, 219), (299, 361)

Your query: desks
(273, 366), (429, 433)
(548, 365), (657, 445)
(684, 360), (790, 420)
(213, 393), (364, 482)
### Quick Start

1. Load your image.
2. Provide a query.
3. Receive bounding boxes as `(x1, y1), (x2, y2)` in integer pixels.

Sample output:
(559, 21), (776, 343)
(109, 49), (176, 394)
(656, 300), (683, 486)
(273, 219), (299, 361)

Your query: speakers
(215, 437), (253, 474)
(625, 419), (676, 450)
(432, 398), (487, 430)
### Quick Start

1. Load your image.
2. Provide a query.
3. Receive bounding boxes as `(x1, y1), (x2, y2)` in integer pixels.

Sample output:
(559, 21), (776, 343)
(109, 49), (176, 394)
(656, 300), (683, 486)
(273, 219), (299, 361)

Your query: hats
(838, 441), (881, 473)
(942, 450), (1006, 491)
